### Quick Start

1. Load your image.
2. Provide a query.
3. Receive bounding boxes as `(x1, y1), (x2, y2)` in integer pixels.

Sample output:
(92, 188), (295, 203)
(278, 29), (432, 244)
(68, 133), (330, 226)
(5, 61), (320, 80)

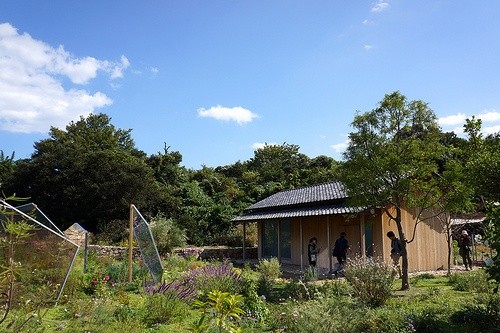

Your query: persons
(335, 232), (353, 268)
(387, 231), (403, 279)
(459, 230), (472, 271)
(308, 237), (322, 277)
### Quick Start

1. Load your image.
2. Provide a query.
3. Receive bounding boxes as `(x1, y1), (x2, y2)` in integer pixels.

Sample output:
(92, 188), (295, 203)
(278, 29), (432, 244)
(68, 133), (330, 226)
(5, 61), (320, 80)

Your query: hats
(460, 230), (469, 236)
